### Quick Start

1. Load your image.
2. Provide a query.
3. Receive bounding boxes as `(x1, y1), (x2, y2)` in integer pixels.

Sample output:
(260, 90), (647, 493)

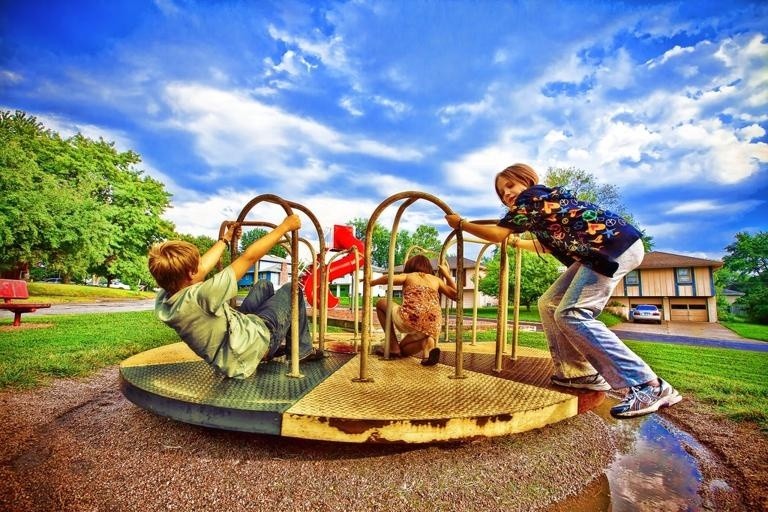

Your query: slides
(300, 224), (366, 310)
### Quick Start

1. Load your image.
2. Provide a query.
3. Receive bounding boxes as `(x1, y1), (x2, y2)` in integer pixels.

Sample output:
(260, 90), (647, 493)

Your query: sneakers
(609, 378), (683, 419)
(299, 347), (324, 361)
(550, 374), (612, 391)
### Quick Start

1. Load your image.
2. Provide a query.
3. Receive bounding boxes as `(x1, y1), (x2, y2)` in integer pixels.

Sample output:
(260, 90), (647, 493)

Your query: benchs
(0, 279), (52, 327)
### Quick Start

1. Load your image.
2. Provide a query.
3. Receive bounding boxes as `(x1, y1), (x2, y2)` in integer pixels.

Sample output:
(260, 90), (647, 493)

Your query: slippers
(420, 347), (441, 367)
(376, 345), (403, 359)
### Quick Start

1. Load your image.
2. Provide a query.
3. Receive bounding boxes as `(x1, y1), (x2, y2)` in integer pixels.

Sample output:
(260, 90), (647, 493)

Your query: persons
(441, 162), (682, 420)
(147, 213), (323, 383)
(357, 254), (457, 366)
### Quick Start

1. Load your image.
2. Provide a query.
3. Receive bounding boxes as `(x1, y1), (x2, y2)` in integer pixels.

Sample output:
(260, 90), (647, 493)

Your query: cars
(632, 305), (661, 324)
(110, 282), (130, 289)
(41, 278), (76, 284)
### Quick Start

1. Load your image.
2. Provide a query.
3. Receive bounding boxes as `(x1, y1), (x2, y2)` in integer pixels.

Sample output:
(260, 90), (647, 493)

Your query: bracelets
(510, 236), (520, 249)
(217, 237), (230, 252)
(457, 217), (464, 231)
